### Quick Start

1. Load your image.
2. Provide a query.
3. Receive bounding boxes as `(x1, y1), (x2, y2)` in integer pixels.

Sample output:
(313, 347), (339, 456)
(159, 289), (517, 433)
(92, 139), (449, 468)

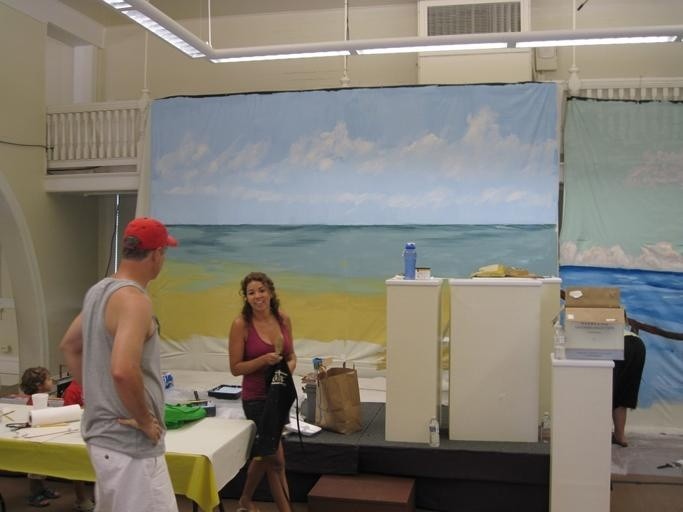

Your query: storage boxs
(552, 288), (628, 362)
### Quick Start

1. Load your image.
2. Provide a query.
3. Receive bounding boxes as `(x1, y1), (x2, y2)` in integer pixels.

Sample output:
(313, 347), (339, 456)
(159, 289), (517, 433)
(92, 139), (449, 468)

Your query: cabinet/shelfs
(548, 352), (615, 512)
(444, 274), (563, 441)
(386, 271), (440, 448)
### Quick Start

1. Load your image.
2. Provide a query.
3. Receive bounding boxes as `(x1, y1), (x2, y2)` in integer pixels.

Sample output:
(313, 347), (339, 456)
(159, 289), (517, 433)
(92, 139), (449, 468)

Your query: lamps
(99, 0), (212, 60)
(210, 0), (683, 64)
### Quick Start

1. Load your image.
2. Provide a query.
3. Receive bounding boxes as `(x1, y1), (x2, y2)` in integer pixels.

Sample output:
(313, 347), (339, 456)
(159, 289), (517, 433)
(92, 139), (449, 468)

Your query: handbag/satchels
(313, 359), (362, 435)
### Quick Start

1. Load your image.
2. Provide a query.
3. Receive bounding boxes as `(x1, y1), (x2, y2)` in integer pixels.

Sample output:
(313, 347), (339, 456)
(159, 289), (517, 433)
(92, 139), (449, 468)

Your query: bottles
(399, 239), (417, 280)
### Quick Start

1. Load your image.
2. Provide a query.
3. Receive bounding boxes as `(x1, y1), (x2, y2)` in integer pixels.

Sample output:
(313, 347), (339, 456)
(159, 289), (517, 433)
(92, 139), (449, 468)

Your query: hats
(122, 216), (176, 251)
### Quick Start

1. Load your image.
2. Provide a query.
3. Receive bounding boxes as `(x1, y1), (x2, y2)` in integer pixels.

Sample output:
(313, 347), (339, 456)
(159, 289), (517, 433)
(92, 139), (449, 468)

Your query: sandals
(40, 485), (60, 498)
(25, 493), (48, 507)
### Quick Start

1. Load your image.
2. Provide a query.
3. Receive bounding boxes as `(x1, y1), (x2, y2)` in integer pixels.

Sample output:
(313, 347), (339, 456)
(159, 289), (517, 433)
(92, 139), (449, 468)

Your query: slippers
(612, 431), (627, 447)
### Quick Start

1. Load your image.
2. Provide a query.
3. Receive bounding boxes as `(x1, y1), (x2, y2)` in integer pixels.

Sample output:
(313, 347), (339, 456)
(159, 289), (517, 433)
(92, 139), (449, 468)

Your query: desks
(0, 403), (256, 512)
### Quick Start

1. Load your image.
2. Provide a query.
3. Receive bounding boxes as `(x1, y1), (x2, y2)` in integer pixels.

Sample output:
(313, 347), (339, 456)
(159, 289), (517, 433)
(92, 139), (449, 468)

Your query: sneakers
(73, 498), (94, 511)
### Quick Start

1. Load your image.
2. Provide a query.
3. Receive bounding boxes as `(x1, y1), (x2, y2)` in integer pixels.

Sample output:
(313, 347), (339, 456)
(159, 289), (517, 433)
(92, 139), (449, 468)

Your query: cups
(29, 393), (50, 410)
(273, 331), (284, 355)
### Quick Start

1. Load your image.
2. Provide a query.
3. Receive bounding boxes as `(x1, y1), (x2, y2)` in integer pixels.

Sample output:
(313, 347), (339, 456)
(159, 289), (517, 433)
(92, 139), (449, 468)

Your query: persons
(612, 325), (645, 452)
(228, 272), (298, 512)
(60, 215), (182, 511)
(63, 374), (92, 509)
(20, 362), (59, 508)
